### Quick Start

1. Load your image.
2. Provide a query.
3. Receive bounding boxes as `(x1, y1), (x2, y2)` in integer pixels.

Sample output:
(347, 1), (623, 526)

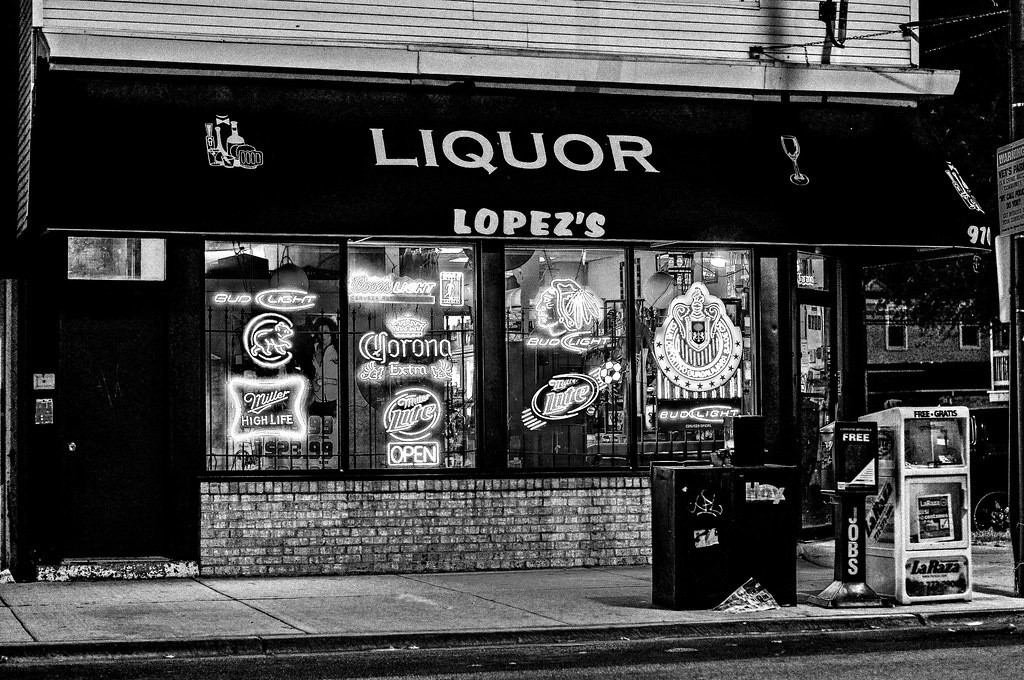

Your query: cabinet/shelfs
(858, 406), (973, 604)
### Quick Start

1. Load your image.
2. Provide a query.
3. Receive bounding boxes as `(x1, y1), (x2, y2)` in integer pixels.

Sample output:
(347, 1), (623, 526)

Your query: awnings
(27, 26), (993, 255)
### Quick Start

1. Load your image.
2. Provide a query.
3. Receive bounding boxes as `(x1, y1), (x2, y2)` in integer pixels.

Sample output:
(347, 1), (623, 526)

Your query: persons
(307, 319), (340, 416)
